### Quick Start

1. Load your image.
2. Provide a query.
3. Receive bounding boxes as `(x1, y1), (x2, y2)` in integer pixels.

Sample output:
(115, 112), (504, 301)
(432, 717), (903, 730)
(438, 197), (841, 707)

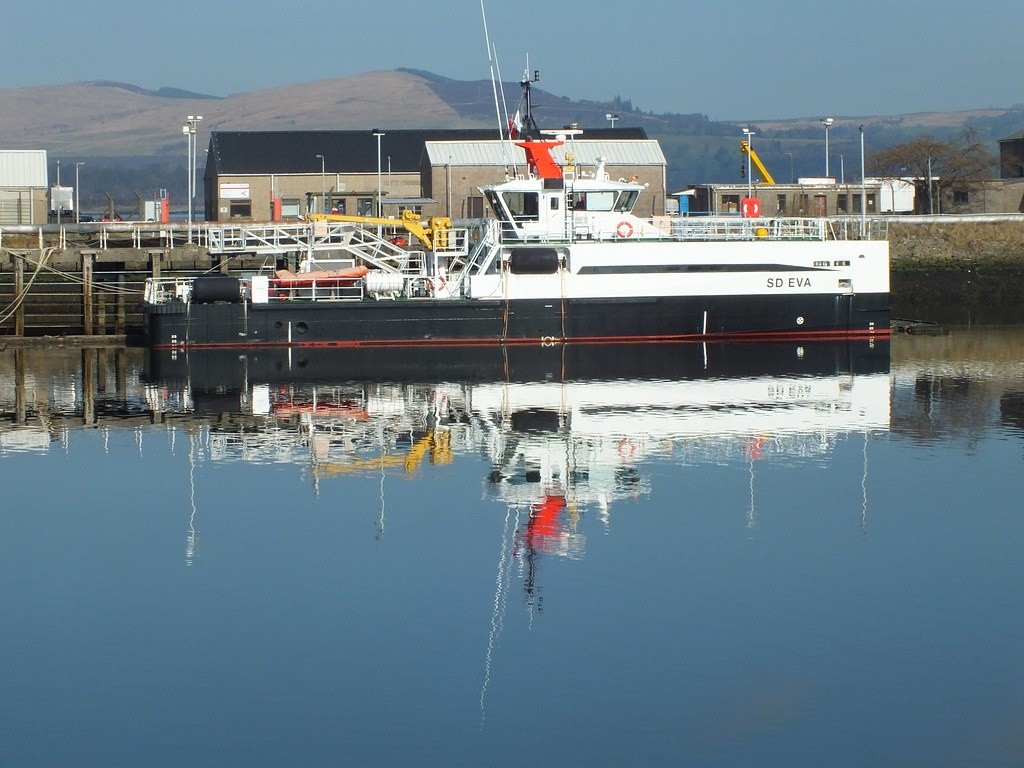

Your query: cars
(79, 212), (120, 222)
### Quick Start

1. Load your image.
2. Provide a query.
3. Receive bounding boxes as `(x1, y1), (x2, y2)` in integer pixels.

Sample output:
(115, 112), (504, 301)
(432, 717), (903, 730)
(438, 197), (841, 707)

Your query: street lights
(605, 114), (619, 128)
(76, 162), (86, 222)
(743, 129), (755, 198)
(821, 118), (834, 177)
(316, 155), (325, 213)
(182, 126), (196, 244)
(837, 154), (844, 184)
(187, 116), (203, 221)
(785, 153), (793, 183)
(372, 128), (385, 218)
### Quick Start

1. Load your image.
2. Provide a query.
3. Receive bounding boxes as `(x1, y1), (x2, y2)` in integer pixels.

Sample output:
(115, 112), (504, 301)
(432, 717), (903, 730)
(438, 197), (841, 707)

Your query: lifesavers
(616, 221), (634, 238)
(429, 276), (446, 292)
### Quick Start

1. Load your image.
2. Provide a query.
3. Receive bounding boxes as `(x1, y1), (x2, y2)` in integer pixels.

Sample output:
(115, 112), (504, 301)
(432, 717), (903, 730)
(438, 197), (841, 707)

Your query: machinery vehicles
(740, 140), (776, 185)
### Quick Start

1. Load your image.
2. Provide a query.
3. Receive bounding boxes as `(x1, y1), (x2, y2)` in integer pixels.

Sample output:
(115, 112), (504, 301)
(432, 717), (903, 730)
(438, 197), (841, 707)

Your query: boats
(137, 0), (891, 349)
(272, 265), (368, 288)
(275, 402), (369, 420)
(138, 335), (891, 711)
